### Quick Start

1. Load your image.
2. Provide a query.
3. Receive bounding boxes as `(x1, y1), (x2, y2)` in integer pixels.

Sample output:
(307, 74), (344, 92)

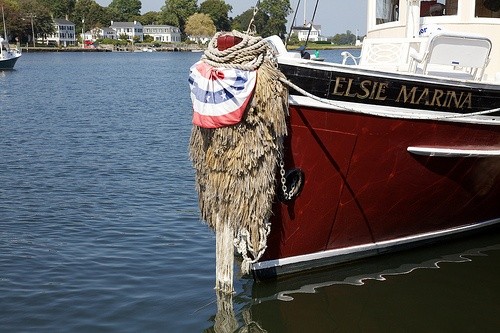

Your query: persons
(297, 45), (320, 59)
(429, 3), (446, 16)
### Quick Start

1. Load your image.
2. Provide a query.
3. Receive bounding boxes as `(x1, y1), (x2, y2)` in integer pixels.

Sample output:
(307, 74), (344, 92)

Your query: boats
(0, 37), (23, 72)
(190, 0), (496, 306)
